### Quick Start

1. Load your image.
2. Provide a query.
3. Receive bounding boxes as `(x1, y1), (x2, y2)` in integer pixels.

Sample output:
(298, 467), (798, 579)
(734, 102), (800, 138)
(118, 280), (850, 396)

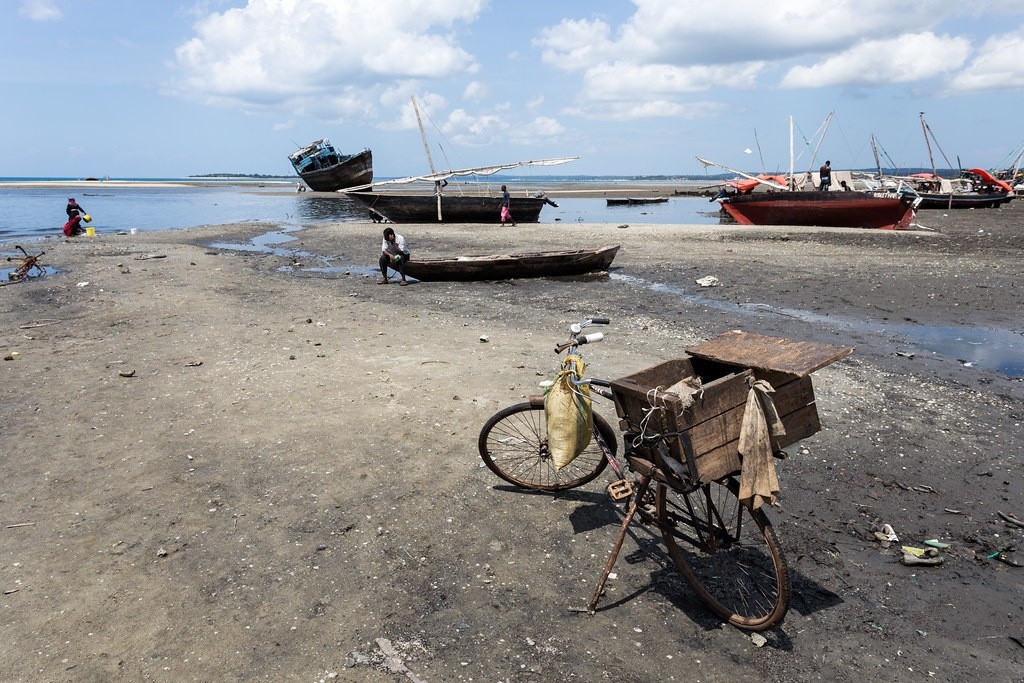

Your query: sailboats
(694, 108), (1024, 209)
(335, 96), (582, 223)
(716, 115), (924, 231)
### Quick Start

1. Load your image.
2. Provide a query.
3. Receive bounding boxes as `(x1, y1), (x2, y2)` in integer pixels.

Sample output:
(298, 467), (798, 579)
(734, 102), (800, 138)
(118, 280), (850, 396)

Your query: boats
(287, 137), (373, 192)
(606, 197), (669, 206)
(385, 245), (621, 282)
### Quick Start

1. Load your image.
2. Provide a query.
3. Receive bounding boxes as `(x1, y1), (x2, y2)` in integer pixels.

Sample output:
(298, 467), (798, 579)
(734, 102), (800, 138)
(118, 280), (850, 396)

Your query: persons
(297, 182), (301, 191)
(63, 198), (86, 236)
(377, 228), (410, 286)
(498, 185), (516, 226)
(838, 181), (851, 192)
(434, 179), (448, 195)
(819, 161), (831, 191)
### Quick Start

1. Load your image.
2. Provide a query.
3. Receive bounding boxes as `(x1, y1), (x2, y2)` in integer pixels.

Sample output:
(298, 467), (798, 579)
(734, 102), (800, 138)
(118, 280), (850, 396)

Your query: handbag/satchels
(545, 354), (593, 471)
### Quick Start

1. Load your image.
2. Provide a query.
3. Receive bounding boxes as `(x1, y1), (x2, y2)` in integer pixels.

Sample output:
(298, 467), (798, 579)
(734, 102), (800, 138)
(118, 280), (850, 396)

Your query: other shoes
(399, 280), (407, 286)
(512, 222), (517, 226)
(500, 225), (504, 227)
(376, 277), (387, 284)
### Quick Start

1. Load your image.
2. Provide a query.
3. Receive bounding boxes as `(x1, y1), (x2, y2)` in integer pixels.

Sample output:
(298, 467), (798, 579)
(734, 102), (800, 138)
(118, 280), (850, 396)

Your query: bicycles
(478, 318), (790, 632)
